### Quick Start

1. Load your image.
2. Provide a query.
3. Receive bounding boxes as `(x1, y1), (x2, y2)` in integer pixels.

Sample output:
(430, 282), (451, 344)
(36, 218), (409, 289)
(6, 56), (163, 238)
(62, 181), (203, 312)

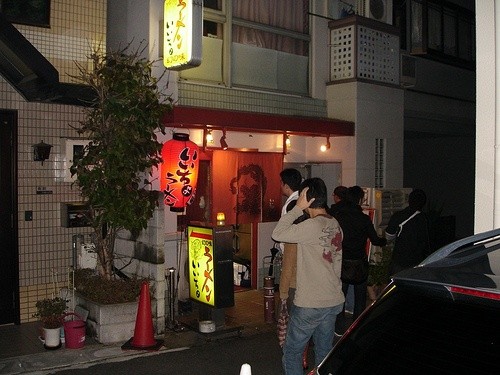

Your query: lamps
(219, 129), (229, 151)
(321, 136), (331, 153)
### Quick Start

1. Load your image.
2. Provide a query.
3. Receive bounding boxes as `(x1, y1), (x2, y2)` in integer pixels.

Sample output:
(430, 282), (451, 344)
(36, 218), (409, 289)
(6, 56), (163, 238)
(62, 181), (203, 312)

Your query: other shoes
(334, 328), (344, 336)
(303, 358), (309, 370)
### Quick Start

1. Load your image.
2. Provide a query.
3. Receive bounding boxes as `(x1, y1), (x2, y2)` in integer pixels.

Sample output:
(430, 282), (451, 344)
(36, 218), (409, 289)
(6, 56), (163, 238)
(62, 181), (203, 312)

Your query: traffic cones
(121, 282), (163, 351)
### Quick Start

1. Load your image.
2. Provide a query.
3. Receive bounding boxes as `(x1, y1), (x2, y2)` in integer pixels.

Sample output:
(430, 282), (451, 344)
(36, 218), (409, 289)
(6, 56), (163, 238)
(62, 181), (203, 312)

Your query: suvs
(308, 227), (499, 375)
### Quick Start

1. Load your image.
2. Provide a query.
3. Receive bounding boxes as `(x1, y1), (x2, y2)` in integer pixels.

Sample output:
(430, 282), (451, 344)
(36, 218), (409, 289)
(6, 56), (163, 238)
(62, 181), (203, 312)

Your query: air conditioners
(399, 54), (417, 87)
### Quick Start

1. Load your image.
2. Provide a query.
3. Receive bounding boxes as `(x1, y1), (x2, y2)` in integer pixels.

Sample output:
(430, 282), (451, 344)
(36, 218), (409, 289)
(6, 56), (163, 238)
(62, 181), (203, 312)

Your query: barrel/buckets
(61, 312), (87, 349)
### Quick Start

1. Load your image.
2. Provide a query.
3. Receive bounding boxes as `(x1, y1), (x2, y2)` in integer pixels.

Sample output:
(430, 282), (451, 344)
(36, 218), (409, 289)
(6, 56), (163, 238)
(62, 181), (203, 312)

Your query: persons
(328, 186), (387, 335)
(385, 190), (431, 277)
(277, 200), (311, 370)
(333, 186), (347, 203)
(279, 168), (303, 254)
(270, 178), (344, 375)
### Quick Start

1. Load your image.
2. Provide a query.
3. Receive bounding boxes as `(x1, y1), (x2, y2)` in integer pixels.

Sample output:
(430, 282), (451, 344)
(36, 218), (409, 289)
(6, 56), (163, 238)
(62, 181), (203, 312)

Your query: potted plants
(62, 37), (182, 349)
(31, 296), (72, 351)
(366, 240), (396, 300)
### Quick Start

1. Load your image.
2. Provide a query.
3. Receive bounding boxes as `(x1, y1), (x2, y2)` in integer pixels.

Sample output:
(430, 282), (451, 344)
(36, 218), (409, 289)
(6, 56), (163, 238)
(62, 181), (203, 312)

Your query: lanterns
(161, 133), (199, 212)
